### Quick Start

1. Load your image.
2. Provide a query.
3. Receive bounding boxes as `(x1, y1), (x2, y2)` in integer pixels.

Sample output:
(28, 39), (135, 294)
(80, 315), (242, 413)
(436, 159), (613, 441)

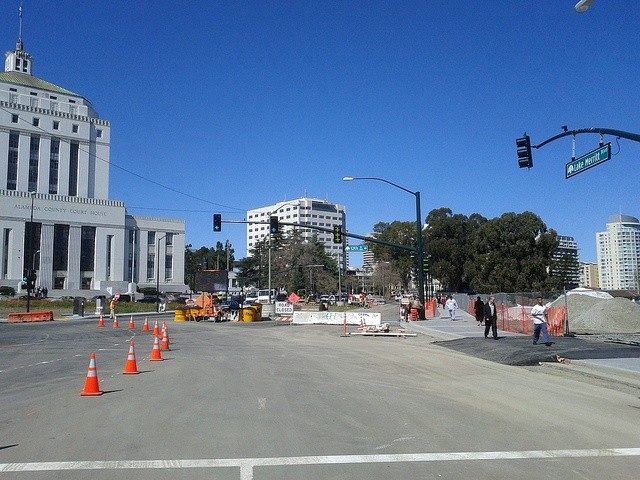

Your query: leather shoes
(495, 337), (498, 340)
(486, 336), (489, 339)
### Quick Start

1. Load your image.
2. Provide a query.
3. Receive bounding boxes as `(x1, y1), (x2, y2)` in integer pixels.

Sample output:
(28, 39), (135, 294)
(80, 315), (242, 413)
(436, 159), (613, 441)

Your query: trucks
(256, 289), (276, 304)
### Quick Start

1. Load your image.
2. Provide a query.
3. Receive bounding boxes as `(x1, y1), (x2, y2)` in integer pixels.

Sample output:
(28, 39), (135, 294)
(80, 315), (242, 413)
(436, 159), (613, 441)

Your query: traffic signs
(343, 245), (369, 252)
(566, 142), (612, 179)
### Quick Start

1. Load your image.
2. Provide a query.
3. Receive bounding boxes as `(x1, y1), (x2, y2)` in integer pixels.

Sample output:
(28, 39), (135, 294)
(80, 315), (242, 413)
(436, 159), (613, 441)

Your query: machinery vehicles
(193, 270), (228, 296)
(196, 289), (221, 322)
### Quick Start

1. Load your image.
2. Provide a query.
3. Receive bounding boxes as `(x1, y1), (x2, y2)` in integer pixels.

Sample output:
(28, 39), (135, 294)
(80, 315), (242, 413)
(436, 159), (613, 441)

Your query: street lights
(157, 232), (179, 312)
(343, 177), (425, 320)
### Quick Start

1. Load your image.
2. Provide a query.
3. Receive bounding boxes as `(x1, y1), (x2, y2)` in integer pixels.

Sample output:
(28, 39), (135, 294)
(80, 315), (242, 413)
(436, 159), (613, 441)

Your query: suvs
(244, 293), (258, 304)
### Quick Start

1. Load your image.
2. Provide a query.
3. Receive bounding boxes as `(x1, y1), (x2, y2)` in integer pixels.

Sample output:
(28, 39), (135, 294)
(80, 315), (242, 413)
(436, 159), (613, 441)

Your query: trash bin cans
(96, 295), (106, 315)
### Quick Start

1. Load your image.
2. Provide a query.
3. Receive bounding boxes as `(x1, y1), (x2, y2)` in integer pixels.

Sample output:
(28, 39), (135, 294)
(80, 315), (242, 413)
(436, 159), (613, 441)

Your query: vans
(321, 295), (329, 301)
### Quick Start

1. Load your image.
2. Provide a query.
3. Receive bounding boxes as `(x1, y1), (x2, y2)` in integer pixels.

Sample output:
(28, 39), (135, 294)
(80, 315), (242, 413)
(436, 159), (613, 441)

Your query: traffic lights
(334, 226), (341, 243)
(214, 214), (221, 232)
(271, 217), (278, 234)
(515, 131), (532, 167)
(22, 268), (36, 289)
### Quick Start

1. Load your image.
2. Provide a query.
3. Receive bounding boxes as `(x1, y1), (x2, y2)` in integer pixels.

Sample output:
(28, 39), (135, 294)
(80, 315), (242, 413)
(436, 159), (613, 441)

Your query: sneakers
(533, 340), (537, 345)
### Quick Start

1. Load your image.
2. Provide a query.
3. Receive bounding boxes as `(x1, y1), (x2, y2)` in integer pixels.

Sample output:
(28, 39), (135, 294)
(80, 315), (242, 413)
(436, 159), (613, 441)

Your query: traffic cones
(128, 314), (134, 329)
(161, 330), (170, 351)
(151, 334), (162, 360)
(113, 315), (118, 328)
(152, 319), (159, 334)
(81, 352), (104, 396)
(121, 340), (140, 375)
(160, 321), (166, 339)
(97, 312), (105, 327)
(142, 316), (149, 331)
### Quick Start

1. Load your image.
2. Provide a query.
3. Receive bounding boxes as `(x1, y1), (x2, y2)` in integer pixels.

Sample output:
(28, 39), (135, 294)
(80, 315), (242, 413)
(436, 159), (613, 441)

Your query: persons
(109, 298), (116, 318)
(445, 296), (458, 321)
(442, 295), (446, 309)
(473, 296), (485, 326)
(530, 297), (551, 346)
(436, 293), (442, 307)
(484, 296), (499, 340)
(36, 286), (47, 298)
